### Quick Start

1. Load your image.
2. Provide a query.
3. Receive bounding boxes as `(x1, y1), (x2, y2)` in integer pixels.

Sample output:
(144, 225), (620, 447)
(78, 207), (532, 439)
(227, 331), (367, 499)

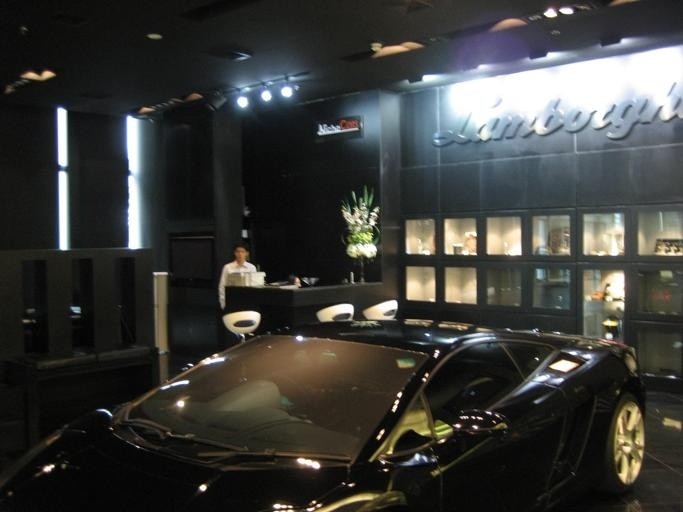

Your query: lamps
(233, 77), (297, 109)
(132, 88), (227, 128)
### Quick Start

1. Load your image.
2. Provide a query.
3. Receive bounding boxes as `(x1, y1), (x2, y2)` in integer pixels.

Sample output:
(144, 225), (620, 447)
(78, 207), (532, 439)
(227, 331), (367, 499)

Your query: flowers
(339, 184), (383, 259)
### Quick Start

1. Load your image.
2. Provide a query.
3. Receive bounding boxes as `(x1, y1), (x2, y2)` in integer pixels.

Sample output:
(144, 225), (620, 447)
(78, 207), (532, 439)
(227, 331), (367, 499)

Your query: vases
(347, 253), (378, 281)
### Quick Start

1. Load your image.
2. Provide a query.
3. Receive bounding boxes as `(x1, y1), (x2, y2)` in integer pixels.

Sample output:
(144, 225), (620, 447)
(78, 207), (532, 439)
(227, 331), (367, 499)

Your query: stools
(359, 297), (400, 323)
(315, 303), (357, 327)
(219, 311), (263, 345)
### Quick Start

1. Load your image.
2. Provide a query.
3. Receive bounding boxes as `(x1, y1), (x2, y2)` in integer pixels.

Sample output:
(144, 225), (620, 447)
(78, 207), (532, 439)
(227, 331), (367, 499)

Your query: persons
(218, 244), (258, 312)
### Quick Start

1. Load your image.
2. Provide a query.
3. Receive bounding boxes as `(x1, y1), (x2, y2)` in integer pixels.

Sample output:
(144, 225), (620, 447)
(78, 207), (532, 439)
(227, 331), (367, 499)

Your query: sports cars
(0, 317), (650, 512)
